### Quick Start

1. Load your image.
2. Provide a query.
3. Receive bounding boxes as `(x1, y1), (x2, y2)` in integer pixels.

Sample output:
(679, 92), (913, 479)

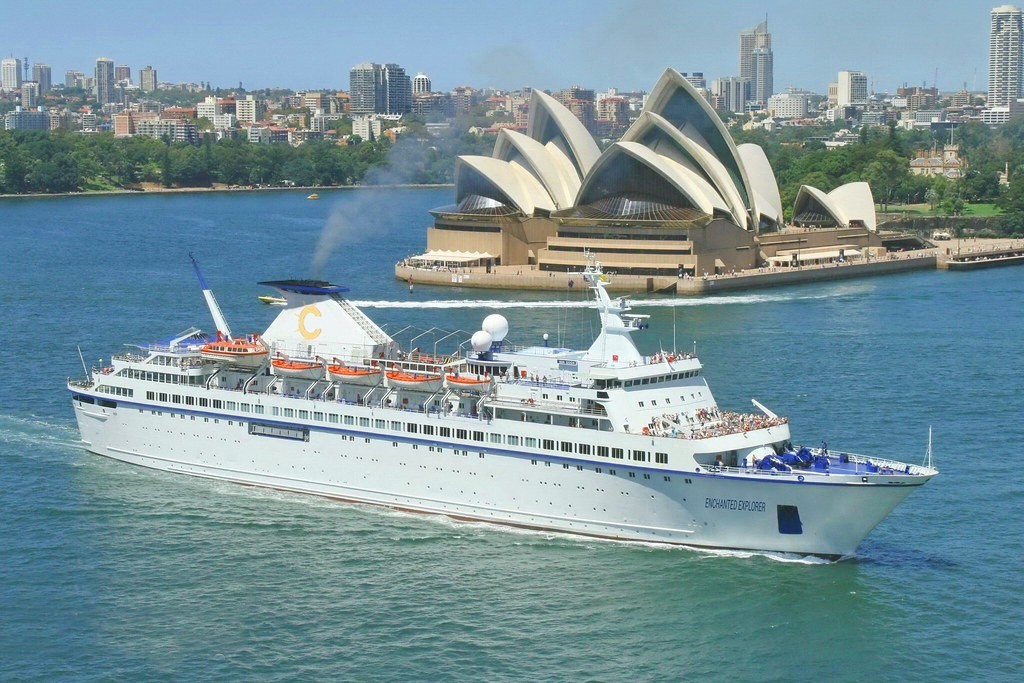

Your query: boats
(258, 295), (287, 304)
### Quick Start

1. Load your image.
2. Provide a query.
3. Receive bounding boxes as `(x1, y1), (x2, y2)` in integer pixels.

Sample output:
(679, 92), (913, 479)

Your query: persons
(73, 329), (492, 423)
(499, 370), (504, 382)
(751, 439), (828, 468)
(536, 374), (540, 387)
(608, 404), (788, 441)
(505, 370), (510, 383)
(542, 376), (548, 387)
(518, 412), (585, 427)
(530, 373), (535, 387)
(391, 212), (1024, 296)
(596, 350), (697, 370)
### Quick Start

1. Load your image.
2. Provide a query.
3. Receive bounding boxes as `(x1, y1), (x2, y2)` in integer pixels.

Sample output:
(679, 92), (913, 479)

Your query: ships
(67, 248), (939, 555)
